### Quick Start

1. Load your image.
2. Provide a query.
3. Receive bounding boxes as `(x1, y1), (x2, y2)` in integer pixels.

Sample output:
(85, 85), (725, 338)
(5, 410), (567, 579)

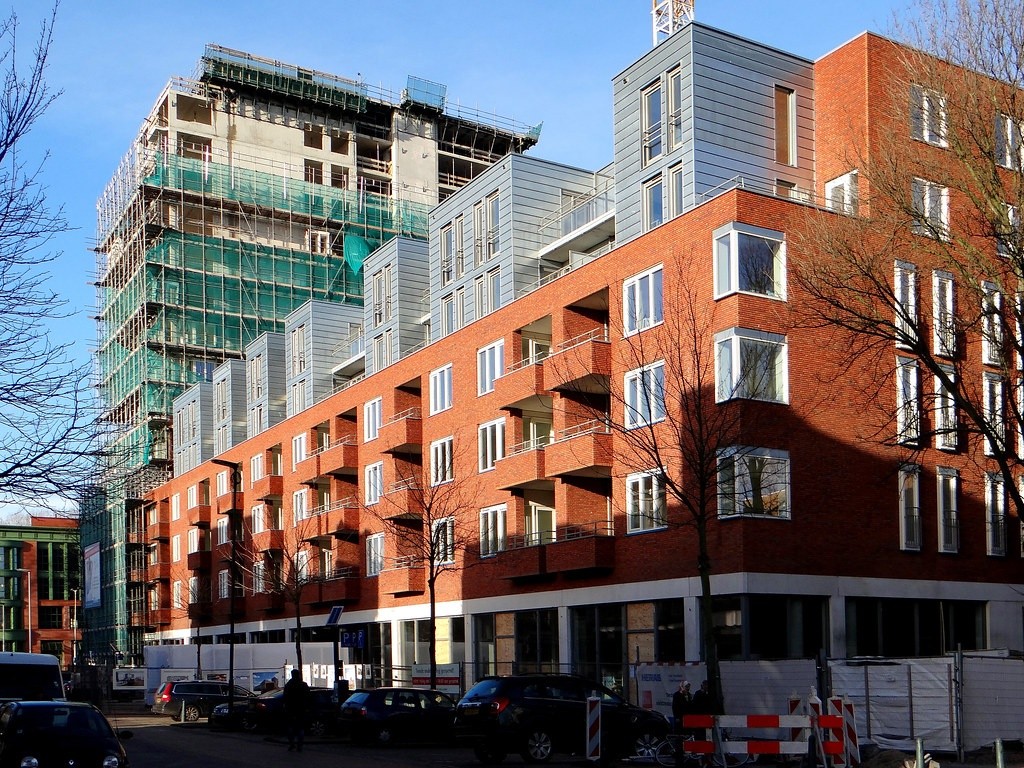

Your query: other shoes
(298, 748), (302, 751)
(288, 746), (296, 751)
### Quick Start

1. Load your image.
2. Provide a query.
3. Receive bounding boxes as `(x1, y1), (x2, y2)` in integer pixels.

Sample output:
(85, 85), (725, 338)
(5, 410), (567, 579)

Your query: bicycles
(656, 722), (750, 768)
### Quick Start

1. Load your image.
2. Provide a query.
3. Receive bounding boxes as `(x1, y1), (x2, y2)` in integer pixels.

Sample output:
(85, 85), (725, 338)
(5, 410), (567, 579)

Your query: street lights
(70, 589), (77, 673)
(211, 457), (239, 724)
(14, 568), (32, 653)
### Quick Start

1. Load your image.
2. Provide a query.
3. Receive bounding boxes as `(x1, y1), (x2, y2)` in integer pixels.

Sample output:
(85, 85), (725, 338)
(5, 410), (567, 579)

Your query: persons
(672, 680), (691, 721)
(692, 680), (710, 715)
(283, 669), (310, 752)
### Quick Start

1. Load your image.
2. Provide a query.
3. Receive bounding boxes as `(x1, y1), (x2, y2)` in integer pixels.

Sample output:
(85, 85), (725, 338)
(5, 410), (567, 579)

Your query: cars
(210, 685), (333, 734)
(337, 686), (455, 747)
(0, 700), (134, 768)
(0, 652), (69, 703)
(153, 680), (256, 724)
(456, 672), (678, 759)
